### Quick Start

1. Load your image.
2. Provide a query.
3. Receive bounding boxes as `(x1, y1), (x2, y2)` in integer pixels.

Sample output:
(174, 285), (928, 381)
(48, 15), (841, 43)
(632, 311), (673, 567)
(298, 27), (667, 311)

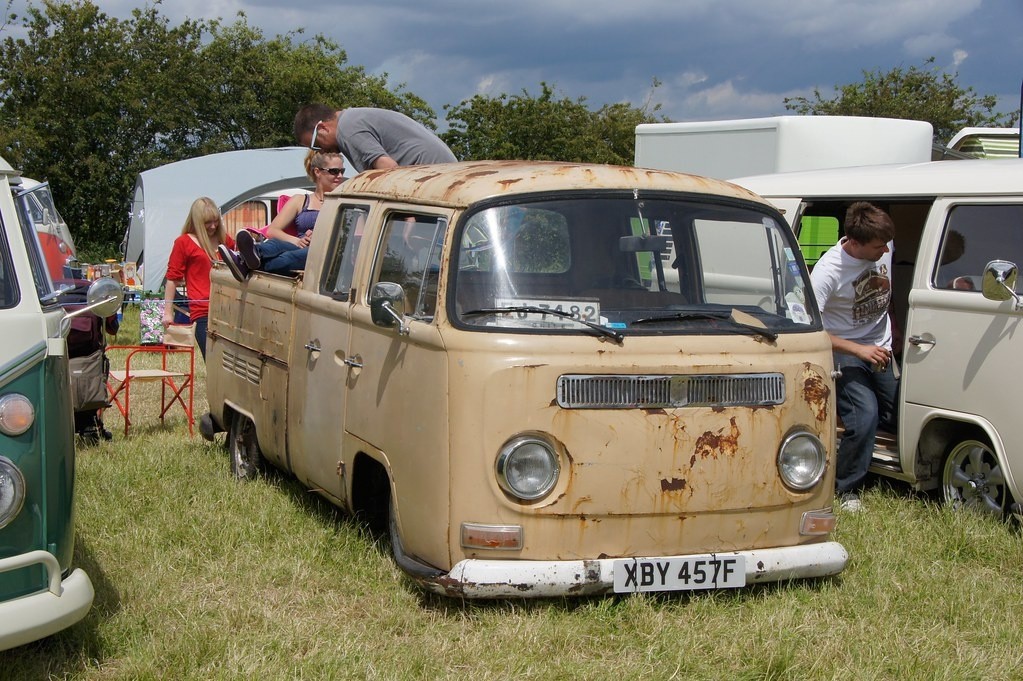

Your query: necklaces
(314, 192), (324, 201)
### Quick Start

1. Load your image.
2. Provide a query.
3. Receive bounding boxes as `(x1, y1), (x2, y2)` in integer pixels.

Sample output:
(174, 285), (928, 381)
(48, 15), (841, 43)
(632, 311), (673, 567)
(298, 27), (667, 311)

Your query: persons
(56, 268), (119, 444)
(295, 103), (469, 268)
(162, 197), (237, 366)
(809, 202), (903, 513)
(218, 147), (346, 282)
(937, 229), (975, 291)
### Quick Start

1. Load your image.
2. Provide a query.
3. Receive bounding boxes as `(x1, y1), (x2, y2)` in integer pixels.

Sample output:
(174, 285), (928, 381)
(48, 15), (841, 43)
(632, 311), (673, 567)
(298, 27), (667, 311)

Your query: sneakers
(218, 244), (249, 282)
(237, 229), (260, 269)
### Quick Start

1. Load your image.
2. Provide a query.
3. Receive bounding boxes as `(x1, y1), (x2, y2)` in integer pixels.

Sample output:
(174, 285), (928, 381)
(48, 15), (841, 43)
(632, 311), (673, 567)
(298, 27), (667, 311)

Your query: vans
(645, 161), (1023, 522)
(10, 177), (76, 290)
(0, 162), (124, 653)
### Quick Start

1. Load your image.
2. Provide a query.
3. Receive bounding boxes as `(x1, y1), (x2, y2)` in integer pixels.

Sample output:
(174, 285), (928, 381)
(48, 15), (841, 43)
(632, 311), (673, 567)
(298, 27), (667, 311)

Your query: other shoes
(841, 492), (864, 512)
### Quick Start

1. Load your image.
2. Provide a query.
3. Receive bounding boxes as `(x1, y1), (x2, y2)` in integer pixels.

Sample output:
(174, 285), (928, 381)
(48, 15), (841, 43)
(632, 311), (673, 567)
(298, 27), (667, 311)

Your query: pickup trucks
(199, 161), (849, 611)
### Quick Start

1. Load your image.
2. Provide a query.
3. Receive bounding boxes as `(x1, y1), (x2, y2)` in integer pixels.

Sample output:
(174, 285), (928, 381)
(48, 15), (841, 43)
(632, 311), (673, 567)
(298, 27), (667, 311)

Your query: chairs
(91, 320), (196, 438)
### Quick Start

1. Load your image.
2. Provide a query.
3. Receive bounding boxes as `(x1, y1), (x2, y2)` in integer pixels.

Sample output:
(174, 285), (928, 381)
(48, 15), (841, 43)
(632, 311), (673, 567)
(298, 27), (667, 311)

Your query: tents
(122, 144), (361, 302)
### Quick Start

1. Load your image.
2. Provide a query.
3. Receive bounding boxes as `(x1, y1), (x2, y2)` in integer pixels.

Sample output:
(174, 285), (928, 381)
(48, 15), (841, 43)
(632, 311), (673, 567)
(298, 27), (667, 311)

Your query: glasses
(318, 167), (345, 175)
(309, 120), (322, 150)
